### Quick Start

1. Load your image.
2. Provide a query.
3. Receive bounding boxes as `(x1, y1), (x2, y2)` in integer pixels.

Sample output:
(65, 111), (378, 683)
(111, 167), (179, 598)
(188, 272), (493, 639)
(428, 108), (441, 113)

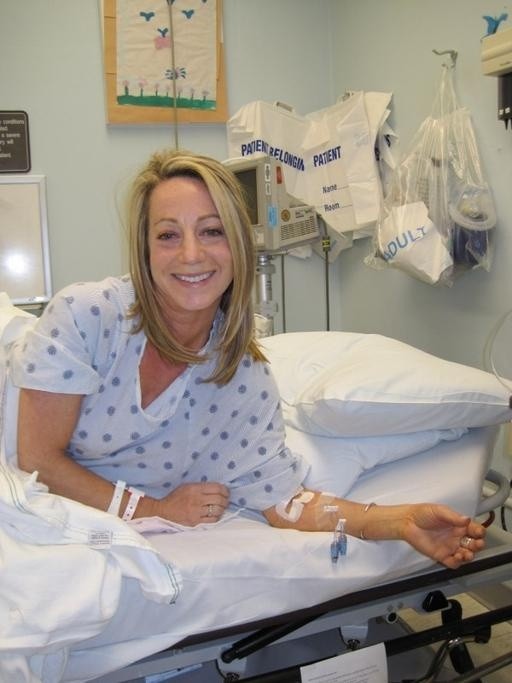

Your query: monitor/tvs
(227, 155), (321, 254)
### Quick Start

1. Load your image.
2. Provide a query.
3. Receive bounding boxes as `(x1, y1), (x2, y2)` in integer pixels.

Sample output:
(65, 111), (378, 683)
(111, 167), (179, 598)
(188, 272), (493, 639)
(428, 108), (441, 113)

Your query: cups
(449, 200), (495, 268)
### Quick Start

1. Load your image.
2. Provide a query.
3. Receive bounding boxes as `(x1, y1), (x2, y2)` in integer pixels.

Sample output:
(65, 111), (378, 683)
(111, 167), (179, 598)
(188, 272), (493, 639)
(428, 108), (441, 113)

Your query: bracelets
(361, 503), (375, 540)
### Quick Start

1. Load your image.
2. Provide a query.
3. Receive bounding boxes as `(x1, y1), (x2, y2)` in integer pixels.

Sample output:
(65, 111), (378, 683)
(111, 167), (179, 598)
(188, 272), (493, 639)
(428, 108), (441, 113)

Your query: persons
(11, 149), (487, 573)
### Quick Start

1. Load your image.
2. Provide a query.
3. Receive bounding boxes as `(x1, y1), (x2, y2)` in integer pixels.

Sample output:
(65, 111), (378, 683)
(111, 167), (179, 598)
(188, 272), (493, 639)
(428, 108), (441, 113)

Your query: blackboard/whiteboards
(0, 176), (52, 306)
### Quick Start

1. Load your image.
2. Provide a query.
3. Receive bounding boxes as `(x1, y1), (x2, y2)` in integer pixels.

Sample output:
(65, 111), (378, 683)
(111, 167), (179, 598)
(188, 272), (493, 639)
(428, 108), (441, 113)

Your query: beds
(0, 421), (512, 683)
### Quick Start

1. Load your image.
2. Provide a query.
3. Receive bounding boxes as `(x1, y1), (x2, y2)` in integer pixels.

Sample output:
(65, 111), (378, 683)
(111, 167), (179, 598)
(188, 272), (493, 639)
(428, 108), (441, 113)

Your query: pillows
(282, 423), (470, 498)
(256, 331), (511, 436)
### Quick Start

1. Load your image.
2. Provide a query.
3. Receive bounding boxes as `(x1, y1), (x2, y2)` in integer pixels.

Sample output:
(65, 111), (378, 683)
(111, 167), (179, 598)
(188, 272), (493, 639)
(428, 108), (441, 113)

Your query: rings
(207, 504), (213, 519)
(460, 534), (474, 549)
